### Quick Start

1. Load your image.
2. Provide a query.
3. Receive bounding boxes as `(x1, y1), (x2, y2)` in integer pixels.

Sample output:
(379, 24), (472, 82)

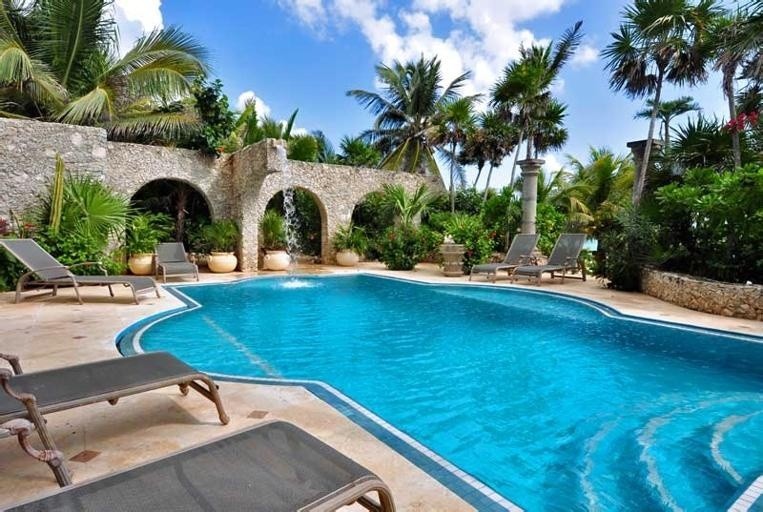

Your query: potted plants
(331, 220), (366, 268)
(258, 209), (291, 270)
(201, 218), (238, 273)
(118, 213), (176, 275)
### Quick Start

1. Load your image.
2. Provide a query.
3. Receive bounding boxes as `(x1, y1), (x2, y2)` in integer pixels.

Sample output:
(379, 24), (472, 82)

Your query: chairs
(468, 234), (541, 283)
(152, 242), (198, 284)
(0, 238), (160, 305)
(0, 417), (396, 511)
(511, 233), (586, 287)
(0, 352), (230, 478)
